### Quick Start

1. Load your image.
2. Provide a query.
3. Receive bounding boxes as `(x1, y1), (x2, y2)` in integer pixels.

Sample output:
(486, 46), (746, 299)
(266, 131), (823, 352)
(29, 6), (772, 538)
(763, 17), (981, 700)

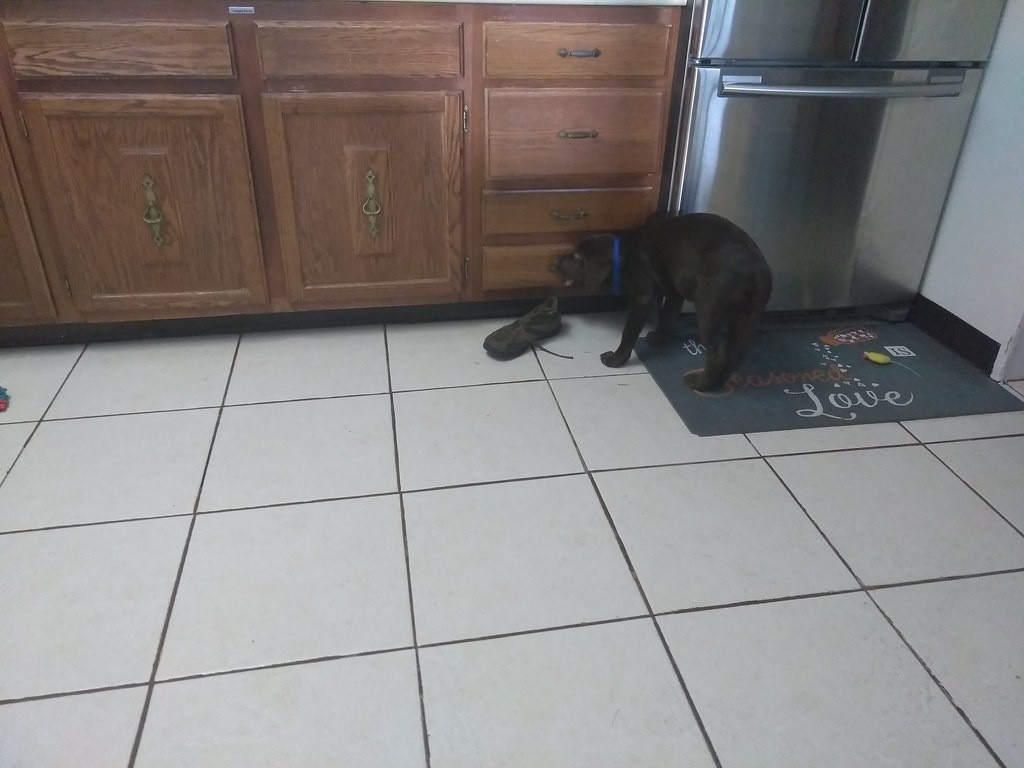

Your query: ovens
(663, 1), (1007, 323)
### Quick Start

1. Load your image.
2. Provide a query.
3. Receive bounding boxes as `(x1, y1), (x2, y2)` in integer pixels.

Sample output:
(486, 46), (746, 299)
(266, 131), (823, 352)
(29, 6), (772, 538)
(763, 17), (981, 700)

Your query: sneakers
(482, 296), (574, 360)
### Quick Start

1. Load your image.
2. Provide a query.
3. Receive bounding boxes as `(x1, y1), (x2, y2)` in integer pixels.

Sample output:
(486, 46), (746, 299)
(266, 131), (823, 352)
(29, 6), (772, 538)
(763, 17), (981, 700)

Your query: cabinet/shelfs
(0, 0), (469, 323)
(468, 3), (683, 318)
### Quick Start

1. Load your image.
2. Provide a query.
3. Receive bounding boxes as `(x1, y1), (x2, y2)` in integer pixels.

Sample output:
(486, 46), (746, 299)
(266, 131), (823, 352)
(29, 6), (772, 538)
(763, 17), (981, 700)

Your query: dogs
(545, 209), (774, 395)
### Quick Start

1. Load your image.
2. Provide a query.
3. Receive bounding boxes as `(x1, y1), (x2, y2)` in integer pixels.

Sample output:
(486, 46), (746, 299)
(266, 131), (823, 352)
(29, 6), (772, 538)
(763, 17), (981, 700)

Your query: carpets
(633, 322), (1024, 438)
